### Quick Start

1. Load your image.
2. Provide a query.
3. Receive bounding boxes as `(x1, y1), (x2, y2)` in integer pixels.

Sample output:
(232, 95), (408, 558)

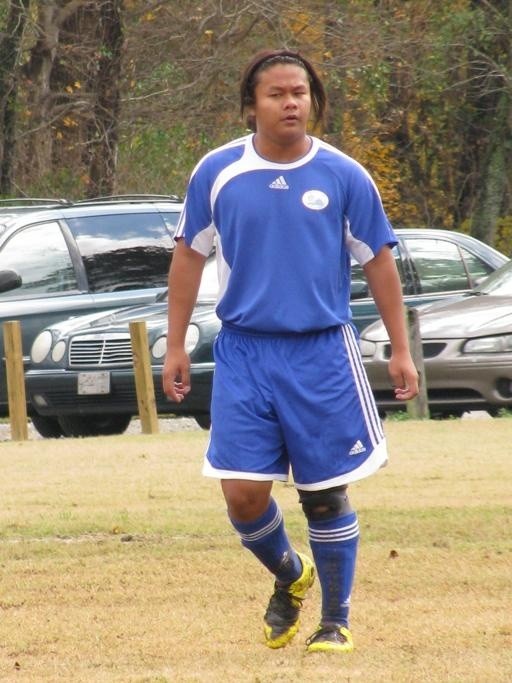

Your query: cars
(358, 258), (512, 414)
(29, 225), (511, 437)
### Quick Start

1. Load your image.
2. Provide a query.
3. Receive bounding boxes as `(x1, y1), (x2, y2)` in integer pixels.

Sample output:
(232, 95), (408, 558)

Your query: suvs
(1, 193), (211, 417)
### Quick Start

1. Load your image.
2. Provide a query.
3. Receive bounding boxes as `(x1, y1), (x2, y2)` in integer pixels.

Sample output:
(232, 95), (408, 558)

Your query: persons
(162, 47), (420, 656)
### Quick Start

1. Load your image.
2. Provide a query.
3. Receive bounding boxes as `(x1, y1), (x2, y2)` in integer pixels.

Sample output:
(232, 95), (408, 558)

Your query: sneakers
(309, 625), (353, 652)
(263, 552), (314, 649)
